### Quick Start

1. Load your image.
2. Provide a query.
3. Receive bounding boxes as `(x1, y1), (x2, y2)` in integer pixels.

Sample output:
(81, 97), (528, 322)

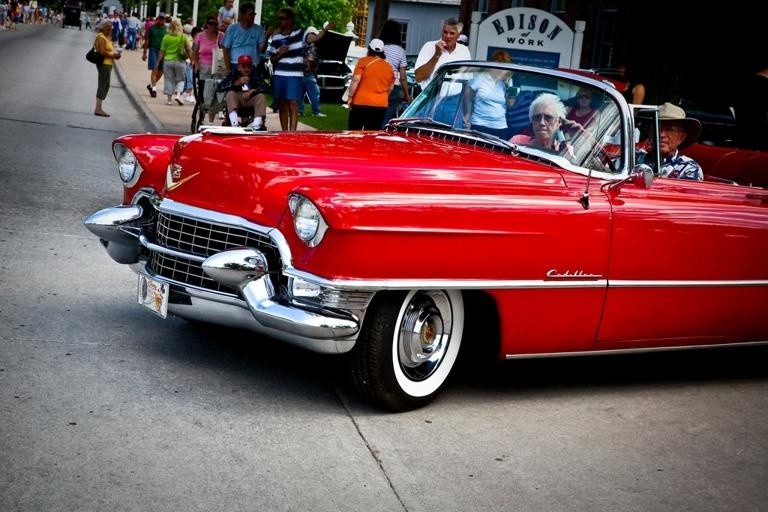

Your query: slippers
(95, 112), (110, 117)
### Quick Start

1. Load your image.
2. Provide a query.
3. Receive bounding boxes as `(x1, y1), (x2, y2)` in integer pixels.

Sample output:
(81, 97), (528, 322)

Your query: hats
(638, 102), (702, 129)
(368, 38), (384, 52)
(238, 55), (253, 65)
(158, 12), (165, 18)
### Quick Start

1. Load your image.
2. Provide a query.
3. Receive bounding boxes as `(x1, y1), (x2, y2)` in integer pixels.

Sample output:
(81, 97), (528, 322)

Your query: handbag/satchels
(303, 58), (316, 77)
(212, 31), (228, 75)
(178, 33), (188, 60)
(86, 34), (105, 64)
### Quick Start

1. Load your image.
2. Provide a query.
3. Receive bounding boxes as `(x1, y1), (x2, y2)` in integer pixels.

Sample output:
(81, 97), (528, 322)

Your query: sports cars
(78, 58), (767, 414)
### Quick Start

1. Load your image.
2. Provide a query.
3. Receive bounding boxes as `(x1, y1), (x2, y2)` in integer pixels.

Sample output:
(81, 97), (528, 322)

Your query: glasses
(207, 22), (219, 26)
(578, 95), (590, 99)
(531, 114), (553, 122)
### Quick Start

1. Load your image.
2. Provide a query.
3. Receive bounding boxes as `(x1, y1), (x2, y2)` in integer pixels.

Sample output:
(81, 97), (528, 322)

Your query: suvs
(61, 5), (81, 30)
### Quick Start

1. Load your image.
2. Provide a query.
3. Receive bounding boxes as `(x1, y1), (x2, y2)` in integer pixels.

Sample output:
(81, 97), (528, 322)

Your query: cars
(263, 29), (354, 92)
(635, 101), (735, 146)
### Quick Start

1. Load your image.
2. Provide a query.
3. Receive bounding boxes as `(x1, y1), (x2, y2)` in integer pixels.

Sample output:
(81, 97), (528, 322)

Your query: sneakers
(168, 92), (197, 106)
(246, 122), (263, 131)
(147, 84), (156, 97)
(311, 112), (327, 117)
